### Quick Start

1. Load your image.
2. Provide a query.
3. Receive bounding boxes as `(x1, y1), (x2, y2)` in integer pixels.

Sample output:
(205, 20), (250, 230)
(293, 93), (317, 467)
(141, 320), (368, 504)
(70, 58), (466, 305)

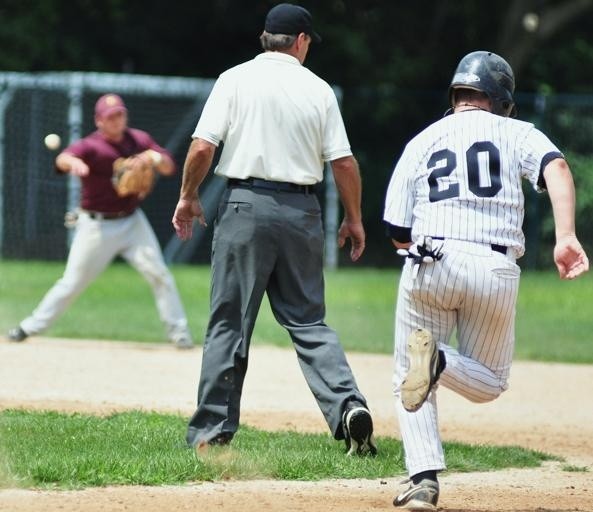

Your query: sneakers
(8, 326), (27, 342)
(172, 331), (195, 349)
(342, 399), (377, 457)
(400, 326), (439, 413)
(392, 479), (439, 511)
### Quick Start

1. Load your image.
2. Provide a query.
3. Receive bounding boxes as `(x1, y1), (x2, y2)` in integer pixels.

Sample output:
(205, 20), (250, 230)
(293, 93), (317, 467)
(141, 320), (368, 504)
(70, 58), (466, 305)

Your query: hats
(94, 92), (128, 121)
(265, 3), (323, 45)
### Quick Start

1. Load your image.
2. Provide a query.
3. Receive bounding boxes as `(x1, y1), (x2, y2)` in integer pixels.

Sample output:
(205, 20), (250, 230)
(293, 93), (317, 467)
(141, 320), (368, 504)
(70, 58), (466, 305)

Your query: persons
(379, 48), (590, 512)
(4, 89), (195, 352)
(170, 2), (378, 460)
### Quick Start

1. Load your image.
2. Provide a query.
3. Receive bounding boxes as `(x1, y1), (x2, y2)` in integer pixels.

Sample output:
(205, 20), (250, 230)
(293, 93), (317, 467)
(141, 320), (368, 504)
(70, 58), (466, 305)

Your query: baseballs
(44, 134), (63, 150)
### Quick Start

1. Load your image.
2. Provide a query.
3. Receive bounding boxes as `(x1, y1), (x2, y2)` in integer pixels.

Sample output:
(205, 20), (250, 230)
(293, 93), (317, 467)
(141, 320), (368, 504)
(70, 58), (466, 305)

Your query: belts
(229, 177), (315, 194)
(492, 244), (506, 255)
(91, 209), (131, 219)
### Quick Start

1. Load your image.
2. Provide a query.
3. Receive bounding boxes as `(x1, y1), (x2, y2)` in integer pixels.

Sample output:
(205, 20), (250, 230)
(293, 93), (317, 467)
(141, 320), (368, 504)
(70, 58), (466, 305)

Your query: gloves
(396, 235), (445, 263)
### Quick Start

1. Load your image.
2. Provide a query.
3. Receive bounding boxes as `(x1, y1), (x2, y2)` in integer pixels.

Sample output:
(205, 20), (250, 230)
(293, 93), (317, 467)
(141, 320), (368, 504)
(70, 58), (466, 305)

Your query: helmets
(448, 50), (515, 117)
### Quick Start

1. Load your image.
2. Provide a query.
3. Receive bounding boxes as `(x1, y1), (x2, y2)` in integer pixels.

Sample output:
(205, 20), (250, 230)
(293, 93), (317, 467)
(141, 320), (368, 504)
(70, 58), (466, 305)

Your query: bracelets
(146, 149), (165, 169)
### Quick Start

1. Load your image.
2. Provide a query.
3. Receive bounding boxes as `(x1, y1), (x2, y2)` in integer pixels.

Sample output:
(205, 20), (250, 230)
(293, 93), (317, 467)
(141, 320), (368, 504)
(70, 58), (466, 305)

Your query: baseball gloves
(111, 151), (156, 199)
